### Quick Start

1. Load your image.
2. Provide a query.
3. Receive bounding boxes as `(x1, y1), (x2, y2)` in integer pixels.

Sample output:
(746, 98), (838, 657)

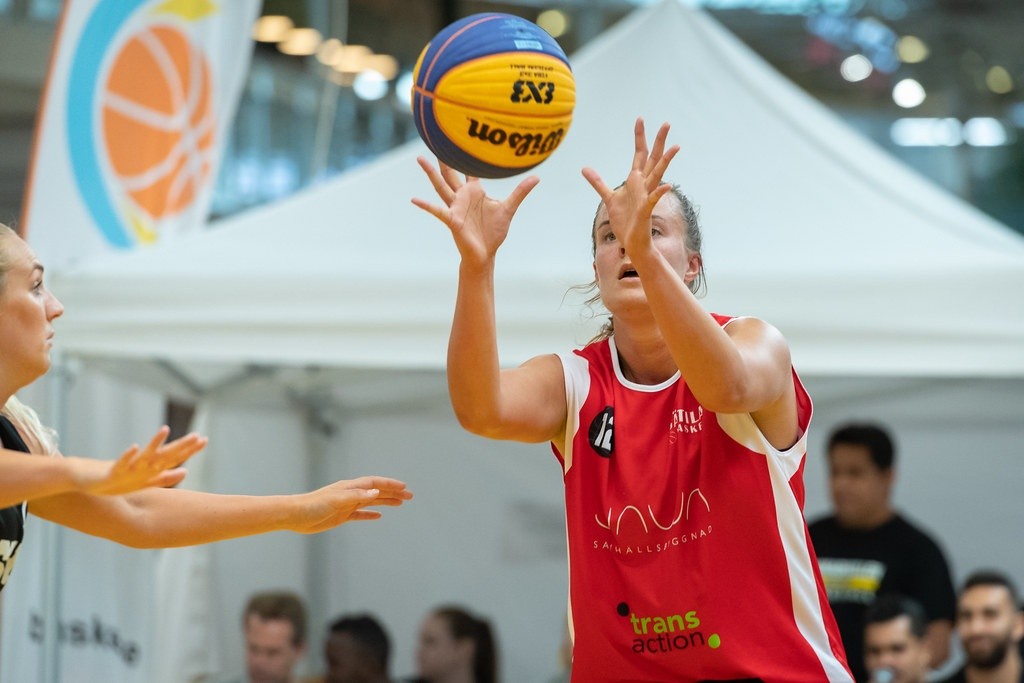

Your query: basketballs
(409, 10), (576, 178)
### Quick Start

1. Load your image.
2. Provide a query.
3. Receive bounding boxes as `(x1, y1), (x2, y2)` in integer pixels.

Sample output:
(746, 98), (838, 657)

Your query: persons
(0, 226), (438, 588)
(241, 588), (497, 683)
(412, 117), (855, 683)
(806, 423), (1024, 683)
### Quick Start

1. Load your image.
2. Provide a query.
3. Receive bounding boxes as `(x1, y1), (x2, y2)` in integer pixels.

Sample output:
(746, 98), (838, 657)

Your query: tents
(0, 0), (1024, 683)
